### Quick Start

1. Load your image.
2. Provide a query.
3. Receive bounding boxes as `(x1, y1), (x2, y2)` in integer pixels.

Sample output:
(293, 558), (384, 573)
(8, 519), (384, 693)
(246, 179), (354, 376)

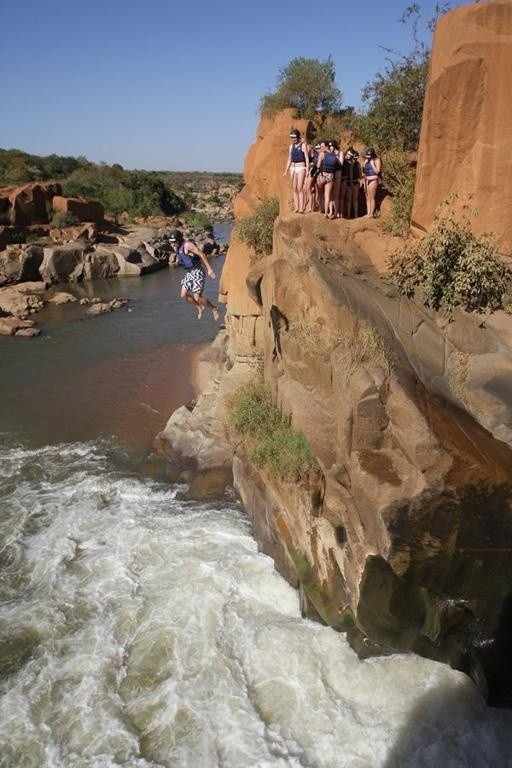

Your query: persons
(166, 230), (221, 322)
(282, 128), (381, 220)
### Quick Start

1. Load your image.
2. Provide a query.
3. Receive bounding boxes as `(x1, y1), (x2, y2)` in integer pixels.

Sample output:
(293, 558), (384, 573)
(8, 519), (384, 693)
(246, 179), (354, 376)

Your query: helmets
(365, 148), (375, 156)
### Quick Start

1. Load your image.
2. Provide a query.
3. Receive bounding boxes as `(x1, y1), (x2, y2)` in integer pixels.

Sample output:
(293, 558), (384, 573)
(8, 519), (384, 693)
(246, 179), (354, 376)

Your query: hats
(289, 129), (300, 138)
(168, 229), (183, 242)
(314, 139), (359, 159)
